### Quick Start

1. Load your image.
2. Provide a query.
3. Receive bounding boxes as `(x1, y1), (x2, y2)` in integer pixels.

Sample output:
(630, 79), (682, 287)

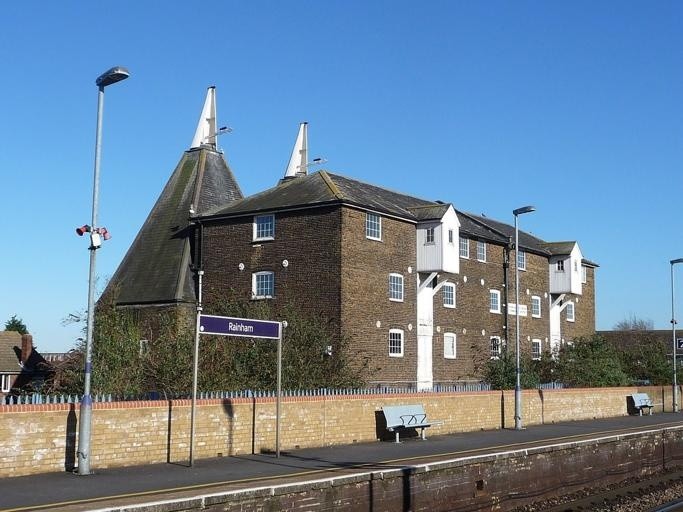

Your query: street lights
(512, 205), (535, 430)
(78, 65), (130, 475)
(670, 258), (683, 413)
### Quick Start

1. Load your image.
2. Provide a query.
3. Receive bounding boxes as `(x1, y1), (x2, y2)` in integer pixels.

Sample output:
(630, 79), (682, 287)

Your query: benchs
(630, 392), (662, 416)
(381, 404), (443, 444)
